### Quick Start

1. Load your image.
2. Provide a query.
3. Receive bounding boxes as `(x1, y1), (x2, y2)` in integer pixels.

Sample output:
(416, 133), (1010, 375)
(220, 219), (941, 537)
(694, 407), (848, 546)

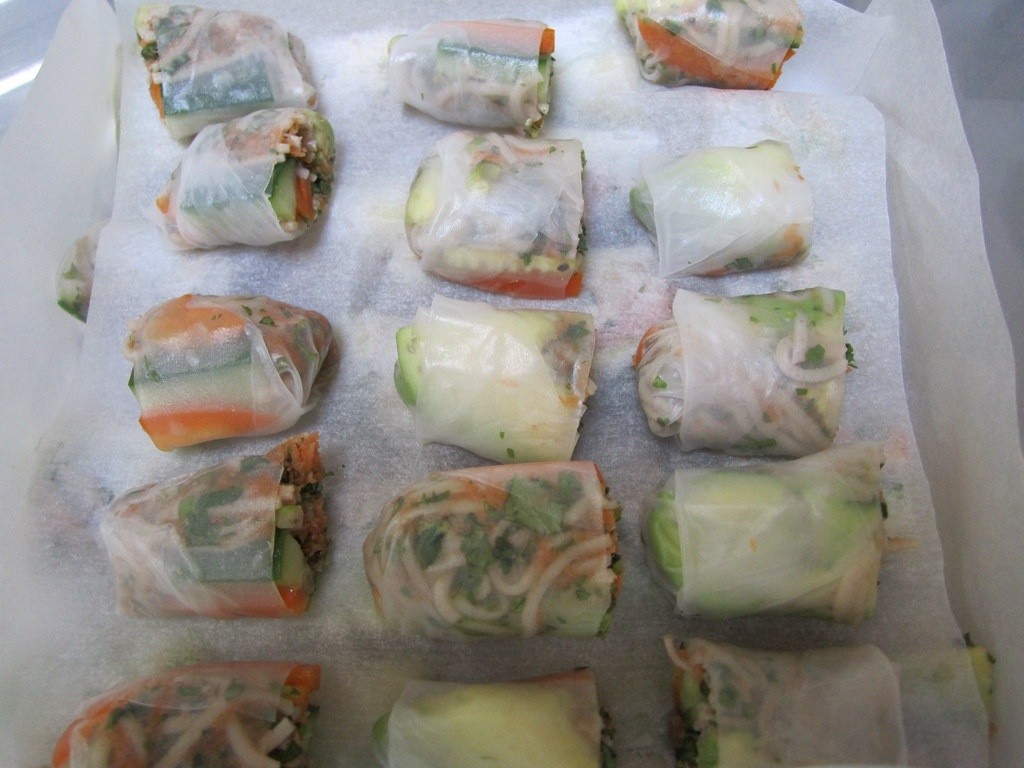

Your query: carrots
(51, 11), (804, 768)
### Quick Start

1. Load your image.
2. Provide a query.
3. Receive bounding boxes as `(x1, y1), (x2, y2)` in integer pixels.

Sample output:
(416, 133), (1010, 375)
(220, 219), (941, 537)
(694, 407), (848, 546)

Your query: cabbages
(647, 285), (996, 768)
